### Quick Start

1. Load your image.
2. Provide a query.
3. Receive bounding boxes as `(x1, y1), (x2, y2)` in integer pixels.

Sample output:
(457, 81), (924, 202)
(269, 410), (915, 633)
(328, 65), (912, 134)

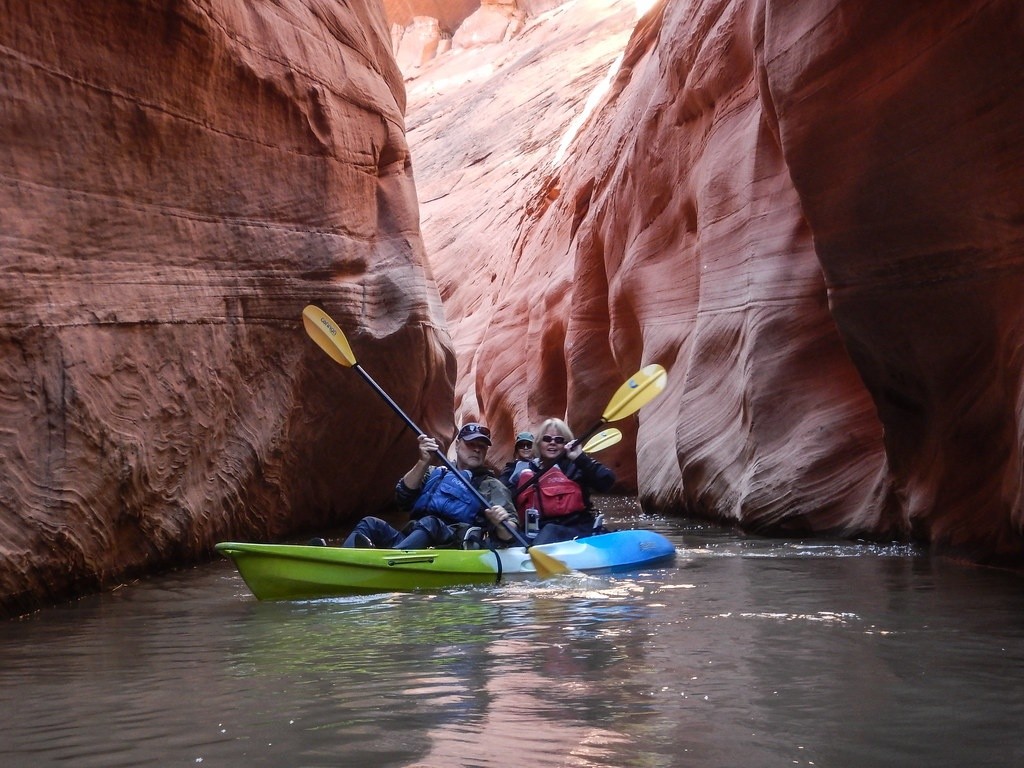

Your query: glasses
(515, 442), (533, 449)
(458, 425), (490, 439)
(542, 435), (567, 444)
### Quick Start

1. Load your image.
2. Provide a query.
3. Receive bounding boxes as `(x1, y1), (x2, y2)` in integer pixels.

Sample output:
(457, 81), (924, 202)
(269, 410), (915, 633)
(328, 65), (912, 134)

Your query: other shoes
(309, 538), (326, 546)
(354, 532), (374, 548)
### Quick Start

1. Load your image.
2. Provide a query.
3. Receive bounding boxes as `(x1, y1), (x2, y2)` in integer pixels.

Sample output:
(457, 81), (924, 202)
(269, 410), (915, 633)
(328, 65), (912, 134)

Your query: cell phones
(524, 509), (539, 538)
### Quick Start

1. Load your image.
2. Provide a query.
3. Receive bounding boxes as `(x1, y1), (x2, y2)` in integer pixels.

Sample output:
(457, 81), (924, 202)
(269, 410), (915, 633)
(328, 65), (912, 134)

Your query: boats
(214, 529), (679, 605)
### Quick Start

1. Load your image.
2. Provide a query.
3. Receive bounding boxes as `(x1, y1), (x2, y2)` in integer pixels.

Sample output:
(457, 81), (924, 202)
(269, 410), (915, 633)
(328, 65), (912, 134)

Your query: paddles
(581, 427), (623, 454)
(299, 302), (573, 582)
(511, 362), (669, 500)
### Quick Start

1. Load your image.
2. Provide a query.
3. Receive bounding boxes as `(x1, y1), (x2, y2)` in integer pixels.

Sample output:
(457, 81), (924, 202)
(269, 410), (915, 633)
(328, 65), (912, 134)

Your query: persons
(307, 423), (521, 549)
(499, 432), (540, 492)
(505, 418), (614, 548)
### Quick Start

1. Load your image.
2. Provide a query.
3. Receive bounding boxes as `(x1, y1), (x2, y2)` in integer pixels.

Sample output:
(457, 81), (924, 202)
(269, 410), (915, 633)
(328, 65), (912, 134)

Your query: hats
(515, 432), (535, 446)
(457, 423), (492, 446)
